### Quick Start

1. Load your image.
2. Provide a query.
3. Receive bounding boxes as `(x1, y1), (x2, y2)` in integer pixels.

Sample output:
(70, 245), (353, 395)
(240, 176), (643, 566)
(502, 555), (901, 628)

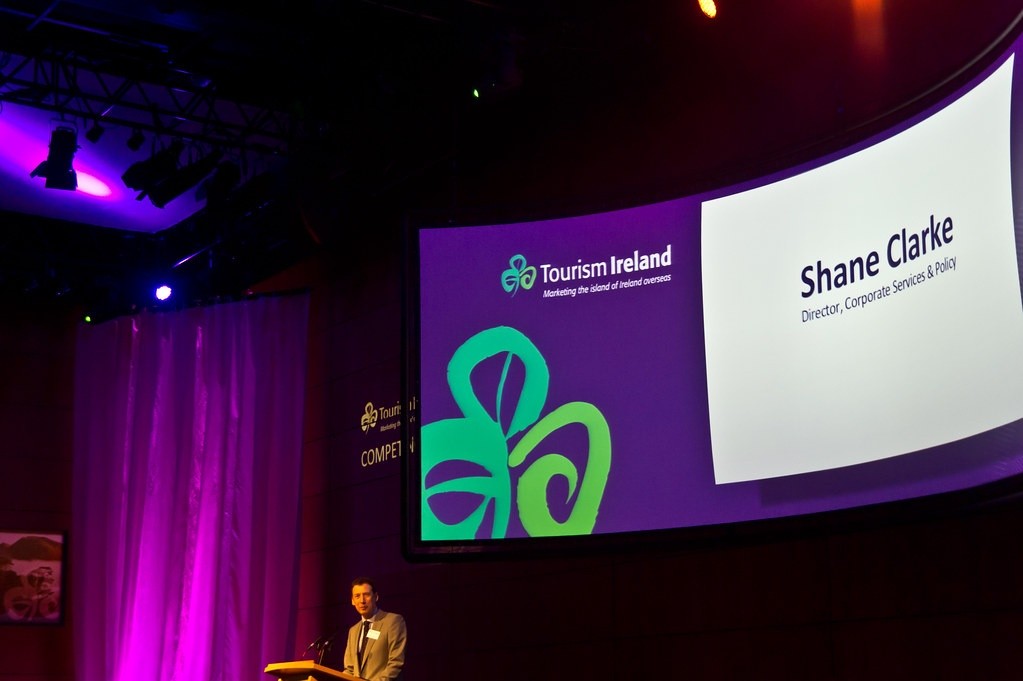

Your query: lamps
(30, 108), (228, 209)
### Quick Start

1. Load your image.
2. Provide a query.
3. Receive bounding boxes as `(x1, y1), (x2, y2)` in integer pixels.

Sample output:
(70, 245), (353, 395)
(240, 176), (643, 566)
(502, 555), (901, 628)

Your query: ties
(360, 621), (370, 666)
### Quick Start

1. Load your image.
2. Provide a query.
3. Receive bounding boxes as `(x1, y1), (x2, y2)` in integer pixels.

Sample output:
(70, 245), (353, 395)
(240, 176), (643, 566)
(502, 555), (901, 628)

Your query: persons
(343, 577), (407, 681)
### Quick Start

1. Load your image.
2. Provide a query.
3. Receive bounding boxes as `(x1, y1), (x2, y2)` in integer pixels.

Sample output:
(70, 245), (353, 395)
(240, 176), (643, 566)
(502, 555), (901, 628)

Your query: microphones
(304, 632), (338, 655)
